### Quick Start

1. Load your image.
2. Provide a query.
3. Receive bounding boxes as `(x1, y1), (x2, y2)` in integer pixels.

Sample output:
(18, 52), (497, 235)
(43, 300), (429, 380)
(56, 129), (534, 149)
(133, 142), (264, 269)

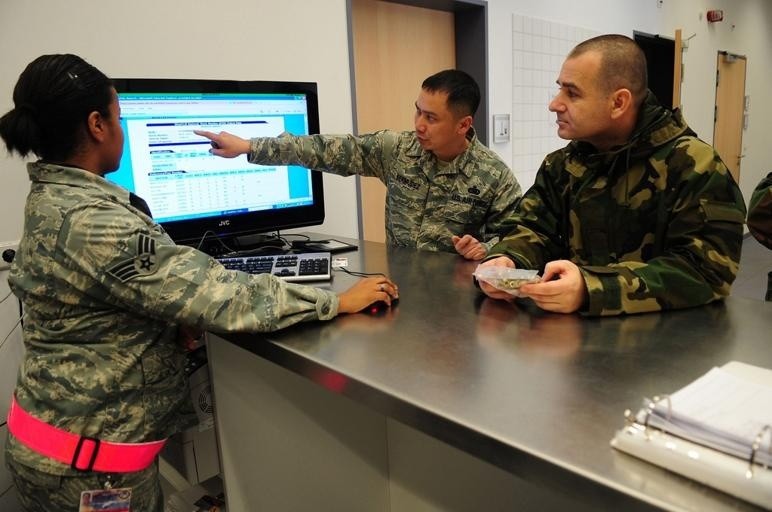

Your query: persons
(472, 34), (748, 320)
(0, 53), (400, 512)
(193, 69), (522, 261)
(747, 171), (772, 302)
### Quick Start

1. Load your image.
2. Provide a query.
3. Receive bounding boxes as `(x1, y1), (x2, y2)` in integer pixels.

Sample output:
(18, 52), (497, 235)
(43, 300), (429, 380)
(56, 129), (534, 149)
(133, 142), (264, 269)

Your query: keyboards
(214, 252), (332, 283)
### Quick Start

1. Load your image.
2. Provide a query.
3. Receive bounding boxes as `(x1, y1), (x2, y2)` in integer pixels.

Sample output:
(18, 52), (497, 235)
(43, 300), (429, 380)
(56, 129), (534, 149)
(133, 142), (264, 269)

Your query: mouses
(364, 295), (398, 313)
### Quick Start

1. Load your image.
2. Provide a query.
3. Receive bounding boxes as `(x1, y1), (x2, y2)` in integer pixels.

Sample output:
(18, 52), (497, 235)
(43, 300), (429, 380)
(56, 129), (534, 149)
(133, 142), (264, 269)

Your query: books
(609, 359), (772, 512)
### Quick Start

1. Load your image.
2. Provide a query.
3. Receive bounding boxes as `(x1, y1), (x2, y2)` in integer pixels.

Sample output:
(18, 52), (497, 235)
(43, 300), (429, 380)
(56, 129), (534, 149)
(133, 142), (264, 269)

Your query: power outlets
(0, 243), (19, 271)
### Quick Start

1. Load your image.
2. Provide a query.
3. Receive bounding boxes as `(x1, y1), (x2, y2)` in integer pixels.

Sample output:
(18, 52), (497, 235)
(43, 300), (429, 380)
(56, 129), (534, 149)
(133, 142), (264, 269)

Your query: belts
(7, 394), (168, 473)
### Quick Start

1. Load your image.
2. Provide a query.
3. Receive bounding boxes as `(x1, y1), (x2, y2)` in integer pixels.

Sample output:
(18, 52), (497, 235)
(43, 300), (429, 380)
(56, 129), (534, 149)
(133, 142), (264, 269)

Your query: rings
(380, 284), (385, 292)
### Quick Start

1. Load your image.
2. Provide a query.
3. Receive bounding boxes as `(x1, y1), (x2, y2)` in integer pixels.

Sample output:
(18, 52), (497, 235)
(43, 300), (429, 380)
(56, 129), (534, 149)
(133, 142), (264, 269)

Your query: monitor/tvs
(100, 78), (325, 256)
